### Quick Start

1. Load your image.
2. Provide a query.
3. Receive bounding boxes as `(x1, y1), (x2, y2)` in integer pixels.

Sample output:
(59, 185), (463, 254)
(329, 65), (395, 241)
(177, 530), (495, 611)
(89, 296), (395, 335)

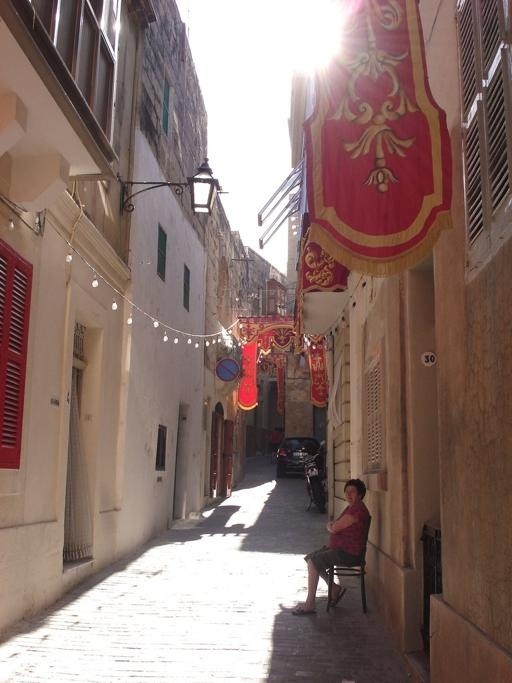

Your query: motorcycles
(297, 452), (328, 515)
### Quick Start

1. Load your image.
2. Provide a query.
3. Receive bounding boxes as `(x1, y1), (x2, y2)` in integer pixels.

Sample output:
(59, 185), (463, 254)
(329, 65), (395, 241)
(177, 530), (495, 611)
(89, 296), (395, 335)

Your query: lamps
(117, 155), (221, 219)
(0, 211), (249, 351)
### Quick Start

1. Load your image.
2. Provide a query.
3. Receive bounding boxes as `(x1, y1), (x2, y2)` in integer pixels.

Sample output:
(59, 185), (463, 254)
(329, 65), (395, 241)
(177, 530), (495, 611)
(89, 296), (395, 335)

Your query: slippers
(328, 585), (345, 608)
(292, 606), (317, 615)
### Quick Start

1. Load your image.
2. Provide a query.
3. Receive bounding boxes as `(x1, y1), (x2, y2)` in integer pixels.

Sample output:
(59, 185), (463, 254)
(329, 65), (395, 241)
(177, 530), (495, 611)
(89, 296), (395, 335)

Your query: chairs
(326, 514), (372, 614)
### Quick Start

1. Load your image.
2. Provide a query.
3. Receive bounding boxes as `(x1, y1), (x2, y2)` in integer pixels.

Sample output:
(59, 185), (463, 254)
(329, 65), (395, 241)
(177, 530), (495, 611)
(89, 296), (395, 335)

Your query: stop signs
(215, 357), (240, 382)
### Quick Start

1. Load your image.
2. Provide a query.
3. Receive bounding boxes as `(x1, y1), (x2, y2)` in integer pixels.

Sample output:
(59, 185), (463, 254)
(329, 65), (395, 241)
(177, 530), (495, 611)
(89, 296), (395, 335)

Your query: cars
(274, 436), (325, 478)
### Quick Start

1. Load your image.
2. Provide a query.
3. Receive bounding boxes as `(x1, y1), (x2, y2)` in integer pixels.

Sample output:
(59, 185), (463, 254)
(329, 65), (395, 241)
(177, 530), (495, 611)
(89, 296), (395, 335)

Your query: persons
(290, 478), (371, 615)
(270, 426), (283, 462)
